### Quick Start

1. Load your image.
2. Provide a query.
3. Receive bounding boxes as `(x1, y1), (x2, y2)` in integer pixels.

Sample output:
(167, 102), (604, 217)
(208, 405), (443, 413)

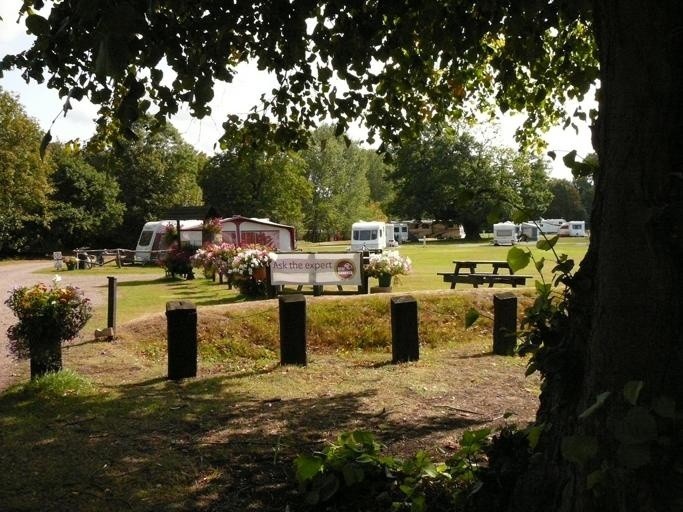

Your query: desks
(449, 260), (518, 290)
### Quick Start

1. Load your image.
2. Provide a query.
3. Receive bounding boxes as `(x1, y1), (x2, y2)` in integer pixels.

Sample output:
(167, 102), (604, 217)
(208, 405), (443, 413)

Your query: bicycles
(72, 246), (93, 270)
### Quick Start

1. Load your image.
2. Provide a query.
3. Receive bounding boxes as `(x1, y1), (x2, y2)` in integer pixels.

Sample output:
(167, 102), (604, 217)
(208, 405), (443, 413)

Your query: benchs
(437, 271), (500, 284)
(468, 272), (533, 286)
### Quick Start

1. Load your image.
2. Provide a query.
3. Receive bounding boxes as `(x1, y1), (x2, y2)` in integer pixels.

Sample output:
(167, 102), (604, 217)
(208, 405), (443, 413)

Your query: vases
(66, 262), (77, 270)
(378, 274), (393, 287)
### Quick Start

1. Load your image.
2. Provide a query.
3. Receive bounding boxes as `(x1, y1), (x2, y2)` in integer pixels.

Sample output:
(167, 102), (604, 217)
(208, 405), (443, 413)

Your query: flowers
(2, 273), (94, 365)
(362, 249), (412, 279)
(155, 218), (272, 282)
(62, 255), (81, 266)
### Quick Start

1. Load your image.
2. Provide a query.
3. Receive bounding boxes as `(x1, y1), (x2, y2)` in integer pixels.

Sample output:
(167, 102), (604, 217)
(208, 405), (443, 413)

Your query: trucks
(131, 216), (272, 267)
(346, 219), (468, 254)
(489, 217), (586, 247)
